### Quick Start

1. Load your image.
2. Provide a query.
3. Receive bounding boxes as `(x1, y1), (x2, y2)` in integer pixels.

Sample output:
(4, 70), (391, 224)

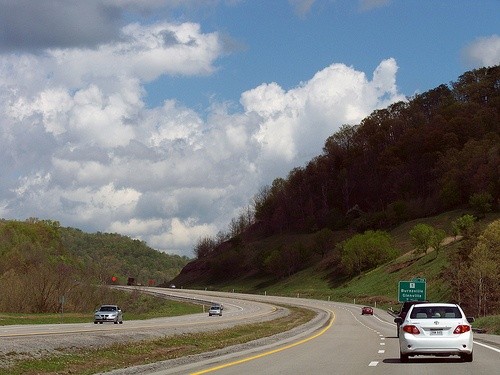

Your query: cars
(169, 284), (176, 288)
(392, 300), (476, 362)
(361, 306), (373, 315)
(208, 304), (223, 317)
(94, 305), (124, 324)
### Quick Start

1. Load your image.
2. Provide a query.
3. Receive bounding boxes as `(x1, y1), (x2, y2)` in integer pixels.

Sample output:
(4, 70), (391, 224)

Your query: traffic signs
(398, 281), (427, 302)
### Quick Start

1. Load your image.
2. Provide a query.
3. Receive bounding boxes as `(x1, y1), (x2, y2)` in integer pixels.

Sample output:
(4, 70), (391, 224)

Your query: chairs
(415, 312), (427, 318)
(444, 313), (455, 317)
